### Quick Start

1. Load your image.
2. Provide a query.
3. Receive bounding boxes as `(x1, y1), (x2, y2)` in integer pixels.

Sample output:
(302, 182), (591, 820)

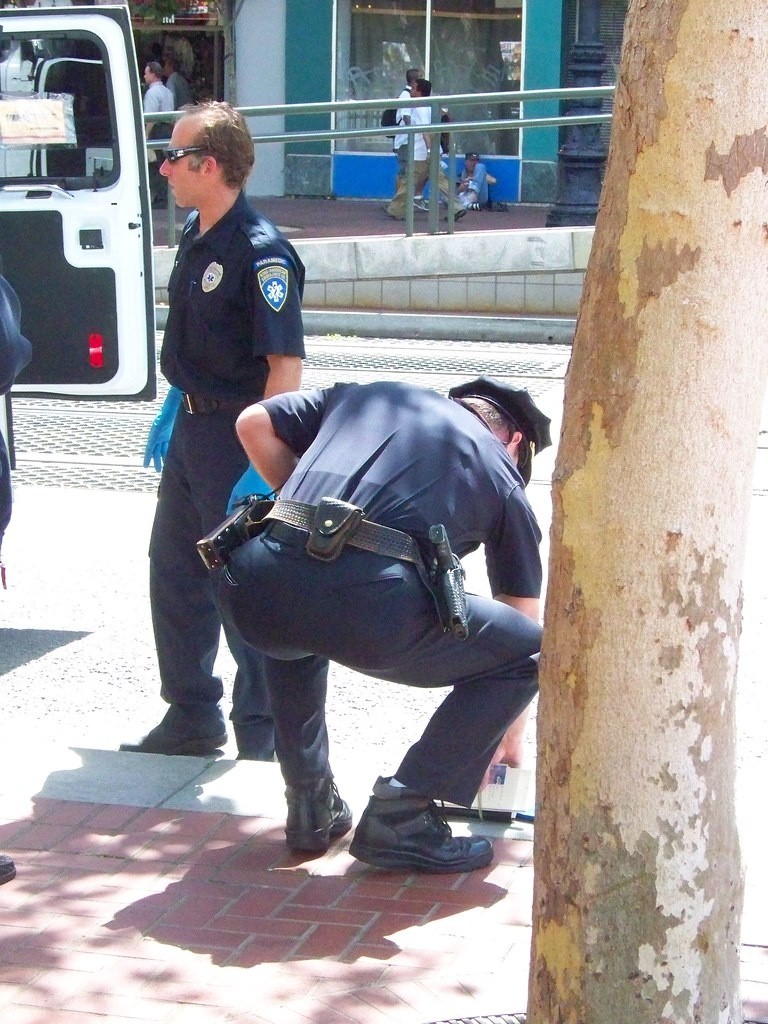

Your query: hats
(465, 151), (479, 161)
(449, 374), (552, 487)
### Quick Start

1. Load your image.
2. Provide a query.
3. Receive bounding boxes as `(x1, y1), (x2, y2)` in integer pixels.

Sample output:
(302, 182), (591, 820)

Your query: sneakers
(413, 198), (429, 210)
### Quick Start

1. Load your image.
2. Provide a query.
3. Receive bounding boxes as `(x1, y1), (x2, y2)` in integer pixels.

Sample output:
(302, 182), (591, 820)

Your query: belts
(180, 392), (264, 414)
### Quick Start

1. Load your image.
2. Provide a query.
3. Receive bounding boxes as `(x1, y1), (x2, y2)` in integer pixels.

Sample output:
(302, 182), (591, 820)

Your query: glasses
(164, 147), (207, 161)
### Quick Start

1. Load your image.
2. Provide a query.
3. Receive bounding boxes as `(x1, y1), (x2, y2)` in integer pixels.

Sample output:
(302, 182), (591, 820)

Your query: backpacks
(381, 97), (400, 138)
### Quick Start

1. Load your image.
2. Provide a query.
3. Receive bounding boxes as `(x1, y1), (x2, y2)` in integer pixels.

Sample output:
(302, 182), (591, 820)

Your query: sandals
(470, 202), (481, 211)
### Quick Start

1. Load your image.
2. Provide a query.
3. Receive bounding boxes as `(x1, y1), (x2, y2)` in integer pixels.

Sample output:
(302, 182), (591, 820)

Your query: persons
(378, 79), (466, 222)
(442, 151), (489, 211)
(163, 59), (192, 103)
(117, 101), (307, 762)
(137, 64), (175, 205)
(393, 69), (433, 211)
(214, 376), (554, 874)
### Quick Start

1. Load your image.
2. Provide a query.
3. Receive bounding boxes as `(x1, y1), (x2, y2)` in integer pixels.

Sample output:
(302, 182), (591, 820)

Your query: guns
(429, 522), (469, 632)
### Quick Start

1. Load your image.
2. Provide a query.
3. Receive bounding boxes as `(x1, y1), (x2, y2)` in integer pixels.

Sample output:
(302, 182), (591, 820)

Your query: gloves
(225, 460), (276, 516)
(144, 385), (181, 474)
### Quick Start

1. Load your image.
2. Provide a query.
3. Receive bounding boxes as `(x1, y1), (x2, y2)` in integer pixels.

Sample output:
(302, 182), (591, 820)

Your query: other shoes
(381, 206), (405, 221)
(444, 209), (467, 222)
(348, 776), (494, 873)
(119, 704), (227, 755)
(284, 778), (352, 853)
